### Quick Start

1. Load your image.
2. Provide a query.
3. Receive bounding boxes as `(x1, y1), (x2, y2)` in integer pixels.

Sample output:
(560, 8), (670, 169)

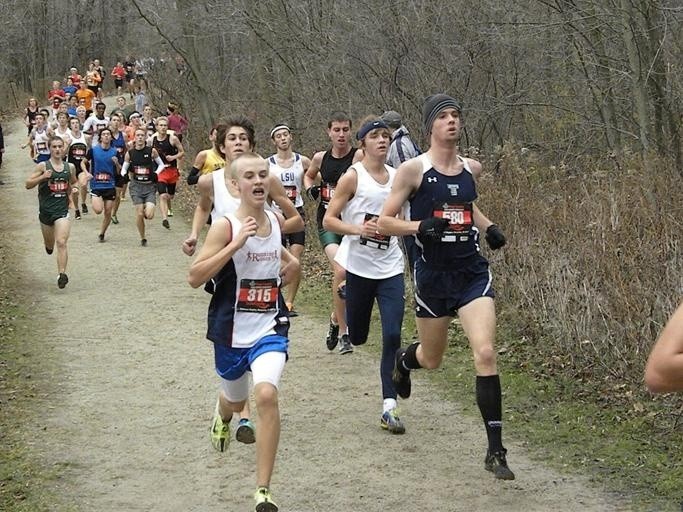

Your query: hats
(422, 94), (462, 134)
(380, 110), (402, 129)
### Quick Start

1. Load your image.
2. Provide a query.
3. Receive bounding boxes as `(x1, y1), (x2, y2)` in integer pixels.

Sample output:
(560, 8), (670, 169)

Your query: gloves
(485, 224), (506, 250)
(419, 217), (451, 236)
(306, 185), (323, 201)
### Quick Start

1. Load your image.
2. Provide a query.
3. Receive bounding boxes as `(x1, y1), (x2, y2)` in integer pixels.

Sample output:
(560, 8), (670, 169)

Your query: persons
(183, 115), (304, 444)
(323, 114), (412, 434)
(263, 124), (322, 316)
(378, 110), (423, 279)
(21, 60), (184, 290)
(188, 152), (291, 511)
(303, 112), (364, 355)
(644, 304), (683, 393)
(187, 126), (226, 225)
(376, 93), (514, 480)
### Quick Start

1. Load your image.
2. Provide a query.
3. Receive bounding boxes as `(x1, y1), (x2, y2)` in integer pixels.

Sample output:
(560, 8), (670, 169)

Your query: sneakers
(111, 215), (118, 224)
(57, 272), (68, 289)
(392, 348), (412, 399)
(210, 410), (232, 453)
(162, 220), (170, 229)
(485, 449), (515, 480)
(285, 301), (297, 317)
(339, 333), (353, 355)
(254, 487), (278, 512)
(96, 234), (104, 242)
(75, 210), (80, 220)
(82, 204), (88, 214)
(380, 407), (405, 434)
(167, 208), (174, 216)
(326, 313), (339, 350)
(235, 418), (256, 444)
